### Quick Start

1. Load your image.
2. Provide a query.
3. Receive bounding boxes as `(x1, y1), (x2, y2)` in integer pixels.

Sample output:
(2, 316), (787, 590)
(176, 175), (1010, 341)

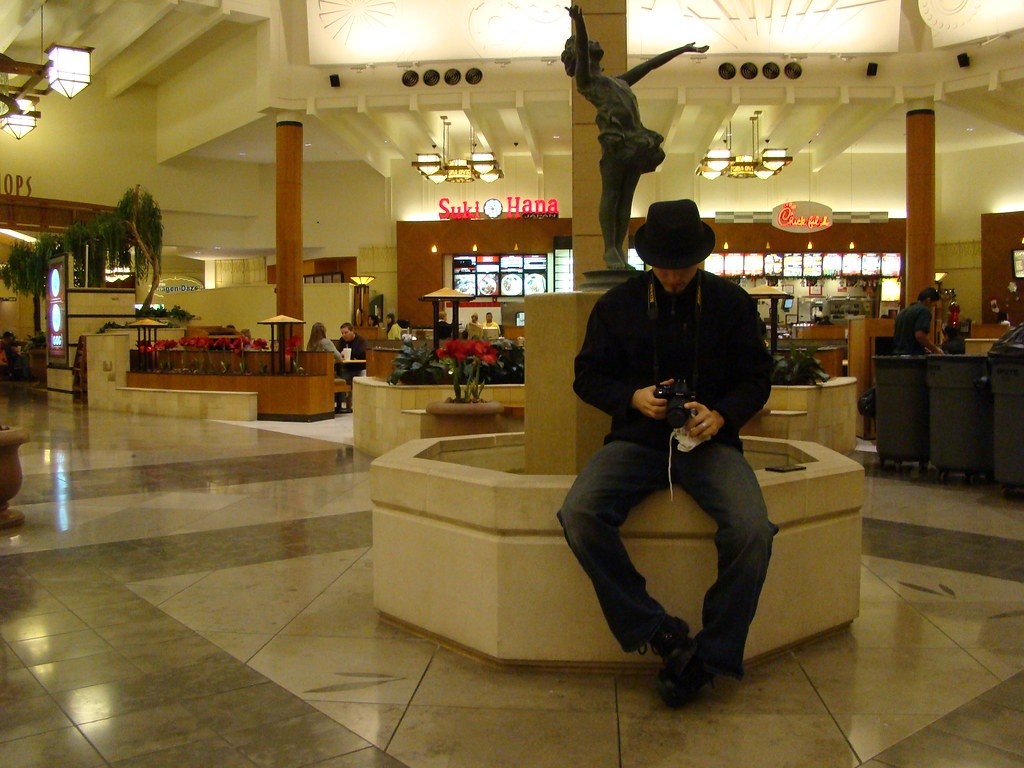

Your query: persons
(557, 199), (775, 709)
(942, 326), (966, 356)
(893, 288), (943, 354)
(337, 323), (366, 376)
(438, 312), (453, 340)
(482, 313), (501, 339)
(0, 332), (24, 379)
(307, 323), (341, 361)
(464, 313), (484, 339)
(273, 341), (279, 352)
(386, 314), (402, 340)
(562, 4), (708, 271)
(369, 316), (379, 327)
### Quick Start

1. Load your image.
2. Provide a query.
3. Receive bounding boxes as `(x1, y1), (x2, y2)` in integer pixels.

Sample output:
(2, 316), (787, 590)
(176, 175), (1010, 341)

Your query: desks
(334, 358), (366, 413)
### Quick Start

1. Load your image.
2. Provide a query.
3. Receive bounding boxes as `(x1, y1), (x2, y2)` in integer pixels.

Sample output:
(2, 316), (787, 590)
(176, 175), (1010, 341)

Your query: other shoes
(636, 612), (697, 672)
(655, 629), (708, 707)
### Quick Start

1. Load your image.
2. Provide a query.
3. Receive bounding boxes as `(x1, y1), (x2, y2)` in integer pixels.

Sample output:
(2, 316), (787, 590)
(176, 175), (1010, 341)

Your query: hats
(634, 201), (716, 270)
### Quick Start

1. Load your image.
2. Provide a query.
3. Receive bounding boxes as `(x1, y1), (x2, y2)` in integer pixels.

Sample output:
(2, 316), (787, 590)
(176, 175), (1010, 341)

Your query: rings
(702, 422), (708, 429)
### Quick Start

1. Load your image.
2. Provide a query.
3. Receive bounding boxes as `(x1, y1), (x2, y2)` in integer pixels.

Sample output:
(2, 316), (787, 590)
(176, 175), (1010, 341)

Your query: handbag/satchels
(857, 387), (876, 419)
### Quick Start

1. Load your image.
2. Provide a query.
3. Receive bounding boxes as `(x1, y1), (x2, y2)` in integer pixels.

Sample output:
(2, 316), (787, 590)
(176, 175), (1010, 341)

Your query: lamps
(0, 4), (96, 140)
(698, 112), (792, 181)
(410, 117), (504, 183)
(350, 275), (376, 325)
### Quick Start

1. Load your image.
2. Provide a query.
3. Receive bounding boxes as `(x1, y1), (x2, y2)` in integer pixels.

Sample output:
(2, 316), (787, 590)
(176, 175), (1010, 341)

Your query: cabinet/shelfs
(848, 317), (897, 440)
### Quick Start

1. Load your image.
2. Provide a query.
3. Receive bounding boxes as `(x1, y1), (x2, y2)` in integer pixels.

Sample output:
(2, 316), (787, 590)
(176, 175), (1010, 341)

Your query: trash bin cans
(929, 354), (991, 479)
(872, 356), (927, 470)
(992, 353), (1024, 483)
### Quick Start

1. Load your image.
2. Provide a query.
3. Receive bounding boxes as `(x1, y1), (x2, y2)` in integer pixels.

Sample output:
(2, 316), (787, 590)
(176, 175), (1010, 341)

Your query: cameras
(653, 378), (698, 427)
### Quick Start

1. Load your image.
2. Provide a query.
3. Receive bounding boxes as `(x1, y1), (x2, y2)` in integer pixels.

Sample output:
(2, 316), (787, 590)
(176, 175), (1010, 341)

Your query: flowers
(137, 334), (307, 376)
(437, 337), (498, 400)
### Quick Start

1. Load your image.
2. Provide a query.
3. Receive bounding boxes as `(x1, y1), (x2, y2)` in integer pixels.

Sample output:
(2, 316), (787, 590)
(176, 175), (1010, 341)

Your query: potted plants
(3, 246), (47, 377)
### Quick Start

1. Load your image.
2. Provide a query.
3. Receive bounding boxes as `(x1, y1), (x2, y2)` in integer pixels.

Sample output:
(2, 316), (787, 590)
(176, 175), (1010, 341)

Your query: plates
(480, 277), (497, 296)
(501, 274), (522, 296)
(524, 274), (546, 295)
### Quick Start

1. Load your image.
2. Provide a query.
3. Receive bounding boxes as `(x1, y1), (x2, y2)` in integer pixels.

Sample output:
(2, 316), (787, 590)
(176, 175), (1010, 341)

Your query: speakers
(330, 74), (340, 87)
(956, 52), (969, 68)
(866, 63), (878, 76)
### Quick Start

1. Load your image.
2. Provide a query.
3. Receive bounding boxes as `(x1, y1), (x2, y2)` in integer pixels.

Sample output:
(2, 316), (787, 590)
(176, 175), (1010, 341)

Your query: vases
(426, 400), (504, 437)
(0, 425), (30, 530)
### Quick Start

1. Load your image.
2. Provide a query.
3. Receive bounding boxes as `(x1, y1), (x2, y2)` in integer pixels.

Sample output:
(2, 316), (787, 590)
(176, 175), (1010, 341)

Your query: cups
(343, 347), (352, 361)
